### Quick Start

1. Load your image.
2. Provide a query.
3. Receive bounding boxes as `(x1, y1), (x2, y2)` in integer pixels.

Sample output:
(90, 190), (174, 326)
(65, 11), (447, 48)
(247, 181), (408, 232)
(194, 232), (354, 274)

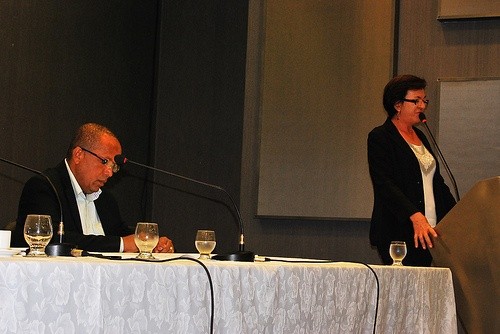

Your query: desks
(0, 256), (458, 334)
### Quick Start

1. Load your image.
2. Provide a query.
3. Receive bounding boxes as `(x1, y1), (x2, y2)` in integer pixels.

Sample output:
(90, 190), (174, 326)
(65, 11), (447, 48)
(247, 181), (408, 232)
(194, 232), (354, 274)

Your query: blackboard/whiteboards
(434, 76), (500, 202)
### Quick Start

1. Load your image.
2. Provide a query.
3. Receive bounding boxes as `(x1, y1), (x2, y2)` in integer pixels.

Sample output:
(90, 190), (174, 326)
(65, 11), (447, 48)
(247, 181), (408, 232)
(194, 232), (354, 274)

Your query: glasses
(78, 145), (121, 174)
(401, 97), (432, 106)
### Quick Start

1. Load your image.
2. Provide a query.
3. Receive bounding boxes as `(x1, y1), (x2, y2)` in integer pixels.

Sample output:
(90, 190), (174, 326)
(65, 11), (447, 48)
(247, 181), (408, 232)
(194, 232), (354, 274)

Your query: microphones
(114, 154), (255, 262)
(419, 112), (460, 201)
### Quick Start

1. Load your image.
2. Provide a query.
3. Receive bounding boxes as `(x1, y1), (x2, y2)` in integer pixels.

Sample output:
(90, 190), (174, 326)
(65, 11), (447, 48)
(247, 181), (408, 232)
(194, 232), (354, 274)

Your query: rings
(169, 246), (173, 250)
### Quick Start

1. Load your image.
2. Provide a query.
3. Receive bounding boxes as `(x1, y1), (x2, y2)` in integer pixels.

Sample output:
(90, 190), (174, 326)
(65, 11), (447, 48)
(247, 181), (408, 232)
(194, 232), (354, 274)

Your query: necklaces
(403, 128), (426, 155)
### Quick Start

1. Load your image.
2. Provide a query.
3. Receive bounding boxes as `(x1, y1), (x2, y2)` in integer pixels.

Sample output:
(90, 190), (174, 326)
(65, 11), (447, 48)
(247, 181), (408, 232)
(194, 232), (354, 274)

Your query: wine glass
(23, 214), (53, 258)
(135, 222), (159, 261)
(389, 241), (407, 267)
(195, 230), (217, 261)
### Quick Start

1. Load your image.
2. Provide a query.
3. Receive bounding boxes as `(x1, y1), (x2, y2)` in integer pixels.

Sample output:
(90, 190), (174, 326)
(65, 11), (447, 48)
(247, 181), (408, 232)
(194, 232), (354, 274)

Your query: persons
(366, 74), (458, 267)
(10, 123), (175, 253)
